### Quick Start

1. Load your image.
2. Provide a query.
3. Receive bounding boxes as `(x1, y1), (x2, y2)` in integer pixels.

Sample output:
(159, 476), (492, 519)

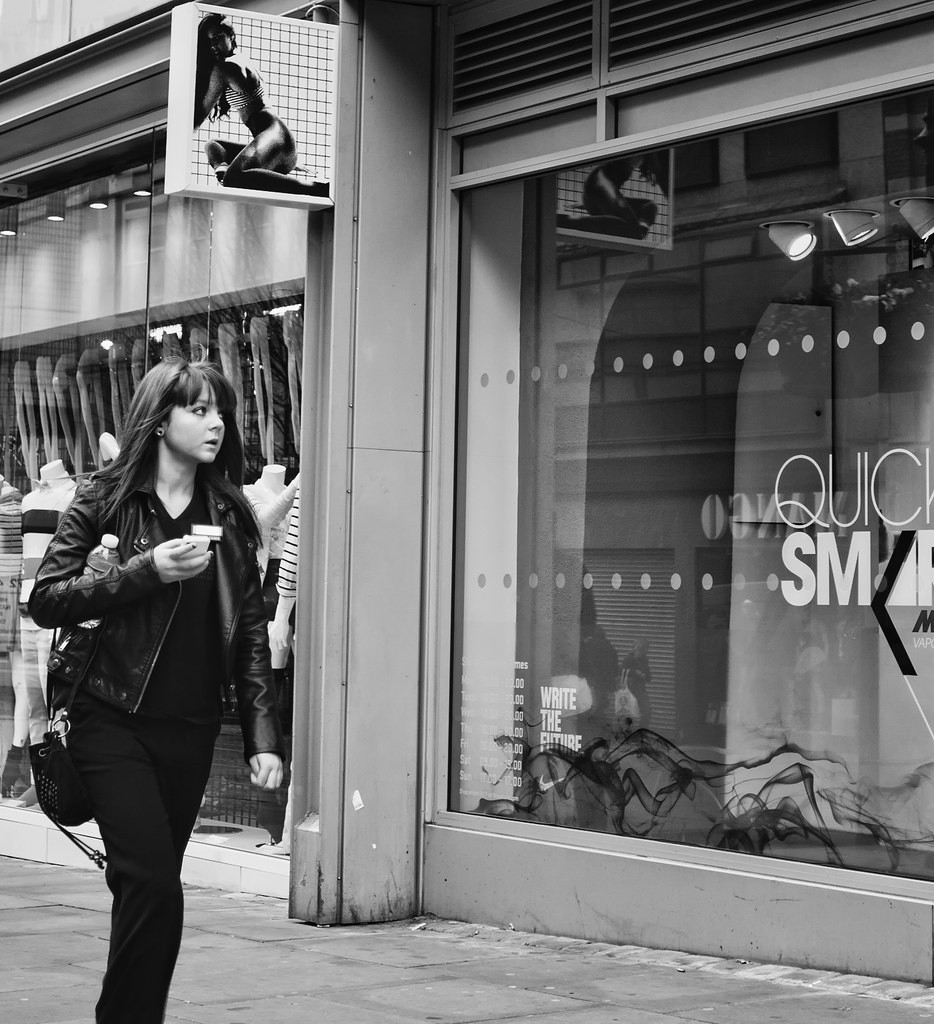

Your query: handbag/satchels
(30, 732), (95, 827)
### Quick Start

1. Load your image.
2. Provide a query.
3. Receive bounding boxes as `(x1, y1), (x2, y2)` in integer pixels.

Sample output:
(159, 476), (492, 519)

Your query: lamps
(830, 211), (880, 247)
(767, 223), (818, 263)
(897, 198), (934, 242)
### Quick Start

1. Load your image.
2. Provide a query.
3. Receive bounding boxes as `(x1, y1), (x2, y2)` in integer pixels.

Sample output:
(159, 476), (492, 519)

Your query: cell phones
(177, 535), (210, 560)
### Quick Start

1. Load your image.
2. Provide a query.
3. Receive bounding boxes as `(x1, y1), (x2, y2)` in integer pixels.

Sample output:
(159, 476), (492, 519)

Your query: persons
(553, 148), (664, 237)
(544, 557), (622, 829)
(28, 358), (285, 1024)
(0, 461), (299, 857)
(618, 640), (655, 746)
(193, 13), (330, 197)
